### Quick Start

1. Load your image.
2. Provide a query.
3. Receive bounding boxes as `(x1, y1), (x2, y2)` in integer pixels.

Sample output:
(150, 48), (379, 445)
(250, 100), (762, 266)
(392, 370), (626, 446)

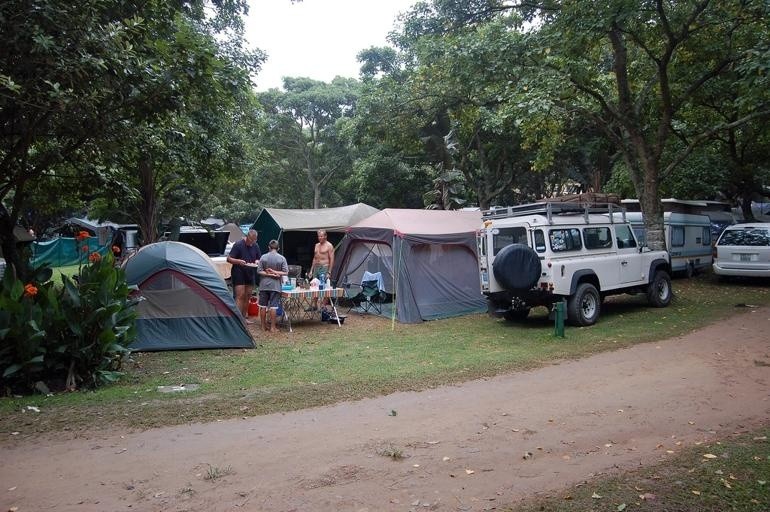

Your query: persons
(256, 240), (290, 332)
(227, 229), (263, 325)
(304, 228), (335, 312)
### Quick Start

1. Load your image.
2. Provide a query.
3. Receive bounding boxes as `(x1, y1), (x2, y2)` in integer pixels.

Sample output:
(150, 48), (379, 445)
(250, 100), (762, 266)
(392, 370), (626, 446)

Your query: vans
(600, 211), (715, 282)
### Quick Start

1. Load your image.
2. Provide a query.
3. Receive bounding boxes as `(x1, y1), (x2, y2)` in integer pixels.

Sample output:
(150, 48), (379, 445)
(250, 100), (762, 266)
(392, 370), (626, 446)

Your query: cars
(711, 221), (770, 277)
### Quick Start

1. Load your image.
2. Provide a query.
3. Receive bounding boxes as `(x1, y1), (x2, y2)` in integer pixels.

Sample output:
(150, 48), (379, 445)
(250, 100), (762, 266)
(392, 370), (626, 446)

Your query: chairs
(286, 265), (302, 285)
(564, 233), (600, 250)
(343, 270), (384, 316)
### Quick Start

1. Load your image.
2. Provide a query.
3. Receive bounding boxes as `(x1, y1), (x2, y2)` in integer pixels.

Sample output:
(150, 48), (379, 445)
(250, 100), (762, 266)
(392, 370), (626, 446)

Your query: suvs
(475, 201), (673, 327)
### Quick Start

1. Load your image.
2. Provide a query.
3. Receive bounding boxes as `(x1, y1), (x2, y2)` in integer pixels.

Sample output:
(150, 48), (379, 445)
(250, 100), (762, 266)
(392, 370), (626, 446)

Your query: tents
(31, 217), (114, 269)
(117, 240), (258, 352)
(247, 202), (381, 278)
(329, 207), (491, 325)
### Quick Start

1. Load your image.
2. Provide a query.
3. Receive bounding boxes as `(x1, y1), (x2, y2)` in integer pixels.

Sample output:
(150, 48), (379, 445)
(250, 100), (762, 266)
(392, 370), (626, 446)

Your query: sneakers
(305, 306), (317, 311)
(318, 306), (327, 312)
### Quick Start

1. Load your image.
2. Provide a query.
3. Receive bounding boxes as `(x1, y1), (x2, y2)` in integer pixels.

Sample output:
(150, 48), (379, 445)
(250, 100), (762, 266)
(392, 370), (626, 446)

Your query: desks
(279, 287), (344, 332)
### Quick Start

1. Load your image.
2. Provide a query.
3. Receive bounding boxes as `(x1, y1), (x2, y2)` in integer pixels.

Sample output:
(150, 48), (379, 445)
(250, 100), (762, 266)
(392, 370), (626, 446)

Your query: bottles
(304, 272), (310, 289)
(319, 272), (326, 289)
